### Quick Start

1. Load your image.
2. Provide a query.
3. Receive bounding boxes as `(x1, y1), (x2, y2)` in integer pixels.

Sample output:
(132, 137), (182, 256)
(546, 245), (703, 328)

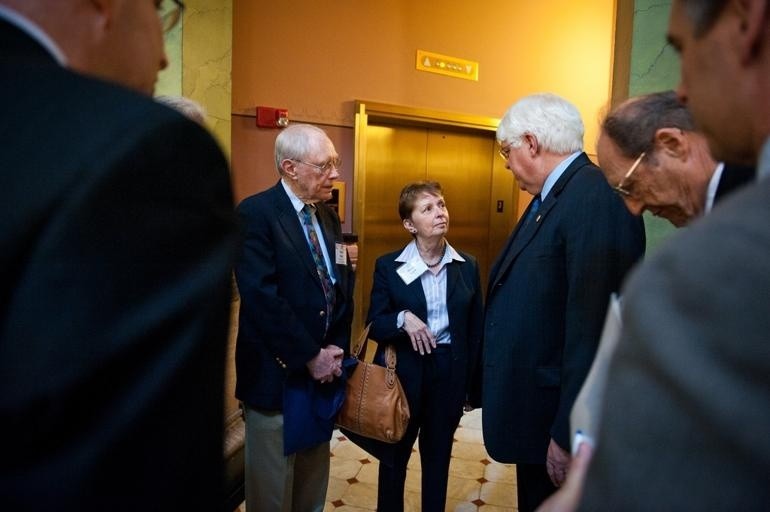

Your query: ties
(300, 204), (336, 339)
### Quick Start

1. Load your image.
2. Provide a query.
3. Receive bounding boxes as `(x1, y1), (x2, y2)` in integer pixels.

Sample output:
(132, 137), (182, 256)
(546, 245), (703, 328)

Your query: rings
(415, 336), (421, 342)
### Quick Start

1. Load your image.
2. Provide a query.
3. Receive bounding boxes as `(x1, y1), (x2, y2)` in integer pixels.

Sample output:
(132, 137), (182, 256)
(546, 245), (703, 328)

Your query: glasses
(497, 142), (514, 161)
(290, 157), (341, 175)
(612, 152), (646, 197)
(161, 0), (184, 33)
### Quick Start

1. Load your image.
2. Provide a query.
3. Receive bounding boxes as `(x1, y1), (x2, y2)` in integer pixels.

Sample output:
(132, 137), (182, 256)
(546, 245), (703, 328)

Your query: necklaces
(415, 238), (448, 268)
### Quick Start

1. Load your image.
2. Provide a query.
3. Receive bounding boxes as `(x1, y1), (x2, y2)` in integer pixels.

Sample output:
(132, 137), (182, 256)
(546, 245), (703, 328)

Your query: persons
(2, 0), (239, 511)
(359, 178), (486, 511)
(570, 0), (768, 510)
(233, 120), (362, 511)
(590, 85), (759, 233)
(475, 92), (641, 512)
(528, 435), (596, 512)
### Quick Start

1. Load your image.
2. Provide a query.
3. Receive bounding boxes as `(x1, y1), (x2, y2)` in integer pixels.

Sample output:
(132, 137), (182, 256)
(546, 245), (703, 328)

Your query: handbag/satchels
(336, 353), (411, 443)
